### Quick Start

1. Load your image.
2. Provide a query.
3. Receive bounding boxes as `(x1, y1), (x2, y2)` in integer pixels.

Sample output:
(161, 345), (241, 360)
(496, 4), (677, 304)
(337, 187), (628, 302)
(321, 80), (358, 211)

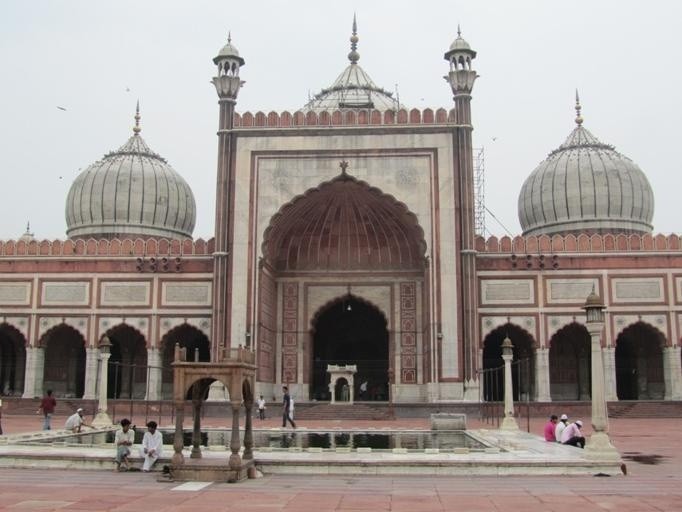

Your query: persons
(258, 394), (266, 420)
(288, 395), (294, 419)
(35, 388), (56, 430)
(543, 415), (558, 442)
(561, 419), (585, 449)
(114, 417), (136, 474)
(62, 405), (98, 434)
(280, 385), (295, 428)
(554, 414), (568, 442)
(139, 421), (163, 472)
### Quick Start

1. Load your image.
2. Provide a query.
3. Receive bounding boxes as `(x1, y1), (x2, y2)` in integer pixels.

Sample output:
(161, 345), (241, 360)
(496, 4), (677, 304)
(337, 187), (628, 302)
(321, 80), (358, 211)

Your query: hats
(561, 414), (583, 426)
(76, 407), (83, 413)
